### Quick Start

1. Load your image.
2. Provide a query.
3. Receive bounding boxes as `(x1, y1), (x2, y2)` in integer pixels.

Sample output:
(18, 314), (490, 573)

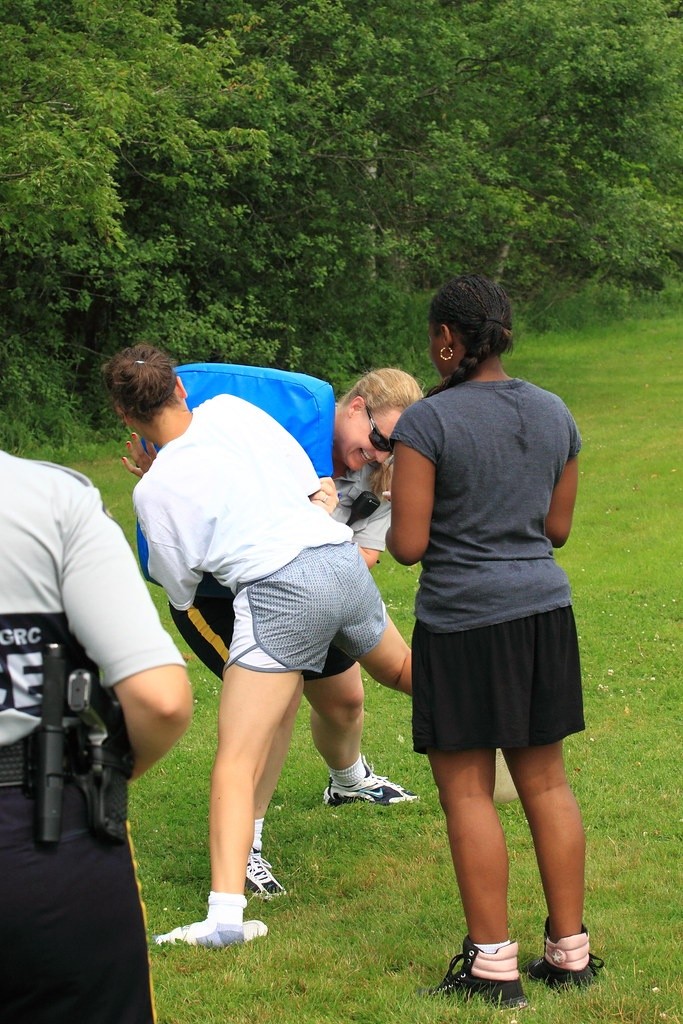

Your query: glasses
(360, 399), (395, 456)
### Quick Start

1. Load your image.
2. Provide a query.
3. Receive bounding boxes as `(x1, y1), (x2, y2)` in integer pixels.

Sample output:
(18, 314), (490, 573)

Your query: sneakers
(242, 847), (287, 904)
(417, 936), (530, 1013)
(523, 916), (598, 992)
(320, 771), (421, 805)
(167, 915), (244, 950)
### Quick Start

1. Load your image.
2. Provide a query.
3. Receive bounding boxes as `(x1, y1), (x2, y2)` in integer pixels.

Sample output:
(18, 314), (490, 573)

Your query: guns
(67, 669), (130, 843)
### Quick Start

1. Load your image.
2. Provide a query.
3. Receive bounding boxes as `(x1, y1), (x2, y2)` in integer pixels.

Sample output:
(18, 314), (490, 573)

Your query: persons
(168, 367), (424, 896)
(383, 276), (605, 1014)
(1, 450), (192, 1023)
(104, 346), (518, 947)
